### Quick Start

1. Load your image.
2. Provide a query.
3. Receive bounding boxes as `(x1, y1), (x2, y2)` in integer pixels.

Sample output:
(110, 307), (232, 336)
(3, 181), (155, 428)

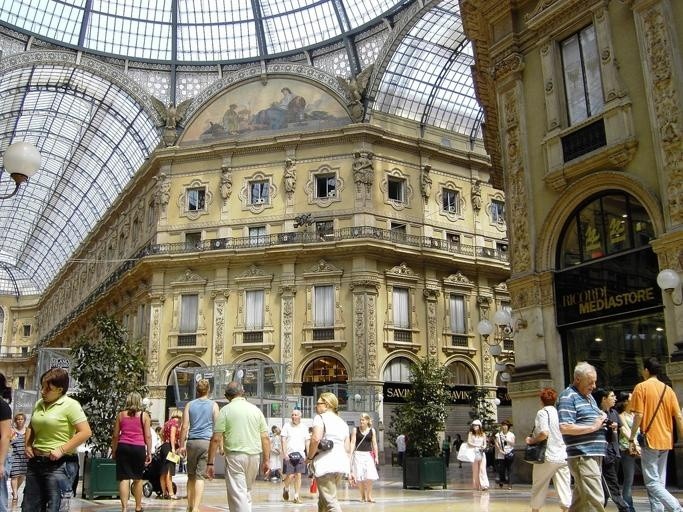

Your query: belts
(29, 456), (78, 462)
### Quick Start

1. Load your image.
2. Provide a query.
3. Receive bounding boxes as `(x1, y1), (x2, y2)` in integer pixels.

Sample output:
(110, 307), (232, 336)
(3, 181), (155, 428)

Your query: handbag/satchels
(457, 442), (475, 463)
(504, 449), (515, 463)
(524, 439), (547, 464)
(637, 434), (647, 447)
(289, 452), (301, 466)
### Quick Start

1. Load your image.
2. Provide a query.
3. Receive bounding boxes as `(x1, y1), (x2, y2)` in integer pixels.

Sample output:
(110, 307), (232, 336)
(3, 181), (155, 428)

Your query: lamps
(371, 394), (384, 412)
(473, 309), (528, 346)
(142, 396), (153, 411)
(655, 268), (682, 308)
(487, 343), (516, 383)
(0, 139), (42, 200)
(349, 392), (367, 406)
(489, 398), (501, 407)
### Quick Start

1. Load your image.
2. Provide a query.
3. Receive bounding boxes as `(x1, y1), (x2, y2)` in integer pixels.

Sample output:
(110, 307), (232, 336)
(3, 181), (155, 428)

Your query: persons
(305, 392), (351, 512)
(442, 356), (683, 512)
(264, 410), (379, 504)
(223, 104), (239, 135)
(112, 392), (182, 512)
(1, 366), (93, 512)
(395, 434), (406, 465)
(177, 378), (271, 512)
(271, 87), (297, 130)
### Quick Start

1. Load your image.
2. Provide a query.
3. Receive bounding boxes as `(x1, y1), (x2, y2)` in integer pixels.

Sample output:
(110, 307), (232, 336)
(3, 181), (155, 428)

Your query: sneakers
(283, 487), (289, 501)
(294, 497), (303, 503)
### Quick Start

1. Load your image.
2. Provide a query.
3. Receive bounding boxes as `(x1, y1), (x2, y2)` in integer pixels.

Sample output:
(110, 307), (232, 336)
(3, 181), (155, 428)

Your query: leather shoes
(163, 494), (182, 499)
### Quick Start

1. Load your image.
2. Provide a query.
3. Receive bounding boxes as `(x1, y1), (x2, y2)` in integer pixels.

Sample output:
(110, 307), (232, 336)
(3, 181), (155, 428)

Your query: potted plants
(68, 314), (155, 502)
(384, 405), (405, 466)
(400, 345), (455, 491)
(466, 386), (500, 469)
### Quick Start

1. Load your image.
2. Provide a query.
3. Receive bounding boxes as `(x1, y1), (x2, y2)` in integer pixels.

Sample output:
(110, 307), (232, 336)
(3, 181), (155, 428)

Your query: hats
(471, 420), (482, 428)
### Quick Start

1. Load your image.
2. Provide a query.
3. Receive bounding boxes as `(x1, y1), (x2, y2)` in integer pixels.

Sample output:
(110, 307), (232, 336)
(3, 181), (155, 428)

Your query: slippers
(135, 507), (144, 512)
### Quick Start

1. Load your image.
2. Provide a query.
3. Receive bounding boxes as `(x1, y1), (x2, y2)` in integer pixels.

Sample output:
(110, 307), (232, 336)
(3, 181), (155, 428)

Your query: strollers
(129, 443), (180, 499)
